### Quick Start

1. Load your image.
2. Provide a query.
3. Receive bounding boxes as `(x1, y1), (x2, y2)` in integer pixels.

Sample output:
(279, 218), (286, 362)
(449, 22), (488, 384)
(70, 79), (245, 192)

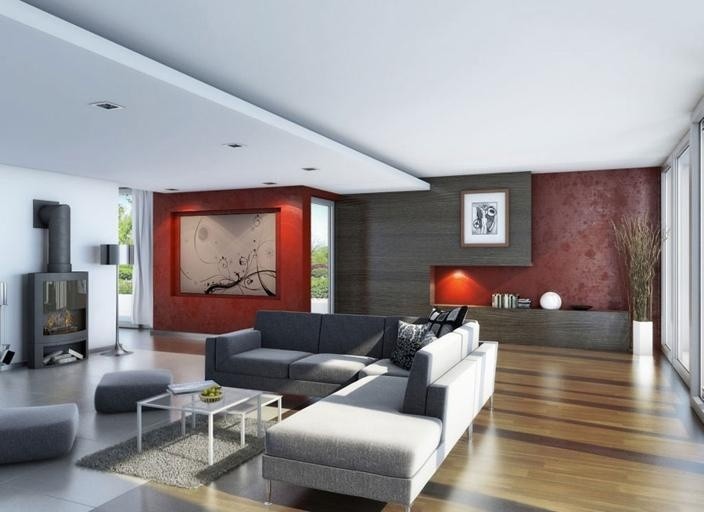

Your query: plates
(570, 305), (593, 310)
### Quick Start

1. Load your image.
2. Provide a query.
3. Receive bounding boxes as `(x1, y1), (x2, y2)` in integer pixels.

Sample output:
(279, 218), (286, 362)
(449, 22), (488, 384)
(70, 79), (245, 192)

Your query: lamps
(100, 244), (133, 357)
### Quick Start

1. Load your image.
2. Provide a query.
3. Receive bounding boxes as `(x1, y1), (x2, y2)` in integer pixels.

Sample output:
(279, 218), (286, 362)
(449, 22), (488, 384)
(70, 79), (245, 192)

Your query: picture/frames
(460, 188), (509, 248)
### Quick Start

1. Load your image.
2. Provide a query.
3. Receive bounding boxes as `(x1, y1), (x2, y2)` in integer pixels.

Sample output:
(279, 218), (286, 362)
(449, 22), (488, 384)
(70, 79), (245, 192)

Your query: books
(167, 376), (222, 395)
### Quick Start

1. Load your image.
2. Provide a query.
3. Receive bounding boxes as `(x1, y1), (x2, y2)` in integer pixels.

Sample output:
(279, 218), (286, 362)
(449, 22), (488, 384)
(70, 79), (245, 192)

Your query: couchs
(204, 310), (498, 512)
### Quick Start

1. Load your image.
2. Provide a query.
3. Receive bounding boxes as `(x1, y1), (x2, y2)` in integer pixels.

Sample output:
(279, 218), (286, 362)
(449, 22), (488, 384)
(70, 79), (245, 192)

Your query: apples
(202, 385), (221, 397)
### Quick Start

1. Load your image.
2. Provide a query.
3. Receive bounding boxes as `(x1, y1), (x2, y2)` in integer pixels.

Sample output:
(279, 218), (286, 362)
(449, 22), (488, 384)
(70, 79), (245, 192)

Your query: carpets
(73, 412), (284, 492)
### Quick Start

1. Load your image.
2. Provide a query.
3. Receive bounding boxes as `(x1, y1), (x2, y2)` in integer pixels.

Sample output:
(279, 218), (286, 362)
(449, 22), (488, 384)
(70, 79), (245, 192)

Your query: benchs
(0, 402), (80, 465)
(94, 368), (173, 414)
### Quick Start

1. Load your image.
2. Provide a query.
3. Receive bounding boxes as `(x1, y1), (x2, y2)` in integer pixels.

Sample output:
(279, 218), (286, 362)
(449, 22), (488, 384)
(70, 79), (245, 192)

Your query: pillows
(390, 305), (470, 369)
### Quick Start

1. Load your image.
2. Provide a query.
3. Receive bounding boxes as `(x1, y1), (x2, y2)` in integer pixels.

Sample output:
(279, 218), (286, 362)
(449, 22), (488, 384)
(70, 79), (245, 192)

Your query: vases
(631, 319), (654, 357)
